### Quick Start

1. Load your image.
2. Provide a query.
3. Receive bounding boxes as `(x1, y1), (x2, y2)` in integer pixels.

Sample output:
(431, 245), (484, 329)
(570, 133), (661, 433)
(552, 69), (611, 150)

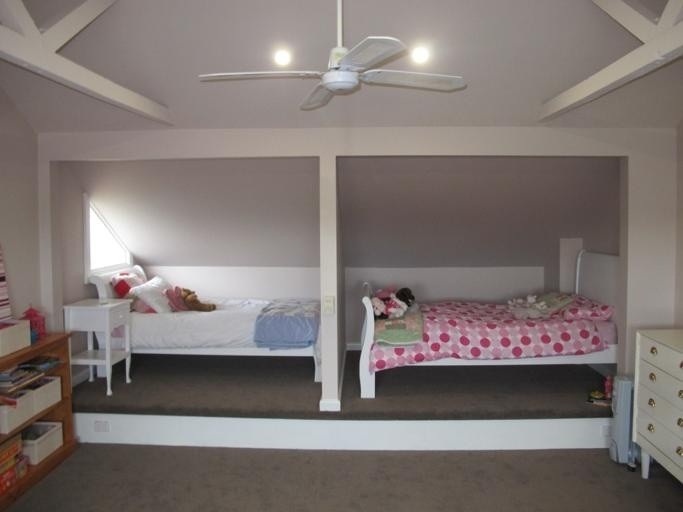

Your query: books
(0, 366), (45, 393)
(0, 433), (30, 496)
(0, 252), (19, 329)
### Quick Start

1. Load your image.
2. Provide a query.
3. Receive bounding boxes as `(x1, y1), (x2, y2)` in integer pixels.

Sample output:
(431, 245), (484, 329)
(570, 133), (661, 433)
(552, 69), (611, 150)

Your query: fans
(196, 1), (468, 108)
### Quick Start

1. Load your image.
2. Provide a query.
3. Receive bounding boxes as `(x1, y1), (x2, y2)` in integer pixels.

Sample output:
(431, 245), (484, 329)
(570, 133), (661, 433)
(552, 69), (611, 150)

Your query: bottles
(605, 376), (612, 399)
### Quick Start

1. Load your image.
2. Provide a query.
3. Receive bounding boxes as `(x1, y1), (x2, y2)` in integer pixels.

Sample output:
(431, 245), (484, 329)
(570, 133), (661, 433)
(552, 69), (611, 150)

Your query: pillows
(557, 292), (614, 321)
(109, 271), (175, 315)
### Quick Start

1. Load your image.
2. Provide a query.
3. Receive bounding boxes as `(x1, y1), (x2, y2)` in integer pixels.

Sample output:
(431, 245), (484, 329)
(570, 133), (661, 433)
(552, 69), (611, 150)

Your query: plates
(590, 391), (603, 399)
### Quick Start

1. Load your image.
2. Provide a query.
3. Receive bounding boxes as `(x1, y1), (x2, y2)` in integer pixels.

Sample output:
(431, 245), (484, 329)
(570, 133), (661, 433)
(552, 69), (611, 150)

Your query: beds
(358, 280), (619, 401)
(88, 264), (322, 383)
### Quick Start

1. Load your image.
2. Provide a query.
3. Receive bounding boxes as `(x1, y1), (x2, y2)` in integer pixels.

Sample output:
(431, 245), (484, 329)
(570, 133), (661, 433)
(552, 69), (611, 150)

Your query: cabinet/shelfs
(630, 329), (683, 483)
(1, 332), (81, 509)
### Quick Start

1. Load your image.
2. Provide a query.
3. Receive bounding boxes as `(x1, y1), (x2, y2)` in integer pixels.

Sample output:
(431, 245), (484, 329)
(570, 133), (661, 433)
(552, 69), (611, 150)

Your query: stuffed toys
(507, 295), (547, 310)
(372, 288), (415, 319)
(181, 288), (216, 311)
